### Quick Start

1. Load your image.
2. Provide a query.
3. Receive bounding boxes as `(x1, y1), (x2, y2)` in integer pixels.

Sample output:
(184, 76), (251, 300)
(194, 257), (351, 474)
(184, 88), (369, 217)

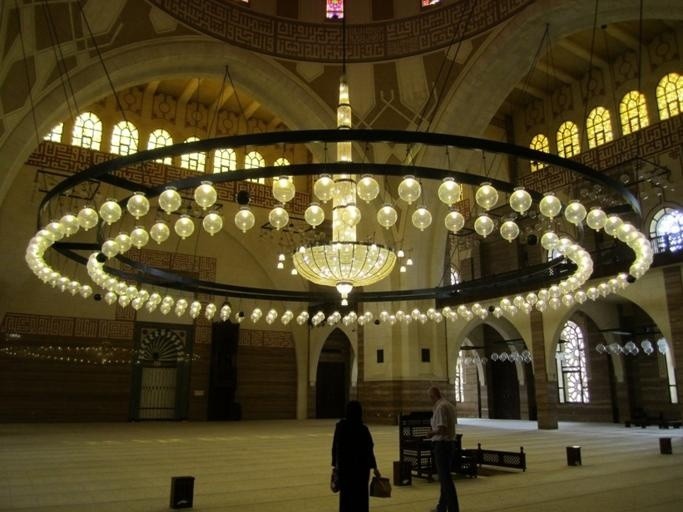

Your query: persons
(329, 398), (380, 512)
(425, 386), (460, 512)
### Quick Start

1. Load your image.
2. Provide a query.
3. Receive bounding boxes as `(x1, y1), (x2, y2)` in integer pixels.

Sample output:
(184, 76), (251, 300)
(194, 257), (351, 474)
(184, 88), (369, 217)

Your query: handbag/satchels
(330, 469), (340, 491)
(370, 476), (392, 498)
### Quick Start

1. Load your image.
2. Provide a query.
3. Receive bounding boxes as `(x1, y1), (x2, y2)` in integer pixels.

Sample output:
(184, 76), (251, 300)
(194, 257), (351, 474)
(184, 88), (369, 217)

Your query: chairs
(399, 409), (464, 483)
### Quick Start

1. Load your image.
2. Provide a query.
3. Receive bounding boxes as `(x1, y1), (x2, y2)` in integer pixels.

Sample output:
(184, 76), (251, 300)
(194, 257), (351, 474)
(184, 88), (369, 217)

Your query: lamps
(24, 3), (656, 329)
